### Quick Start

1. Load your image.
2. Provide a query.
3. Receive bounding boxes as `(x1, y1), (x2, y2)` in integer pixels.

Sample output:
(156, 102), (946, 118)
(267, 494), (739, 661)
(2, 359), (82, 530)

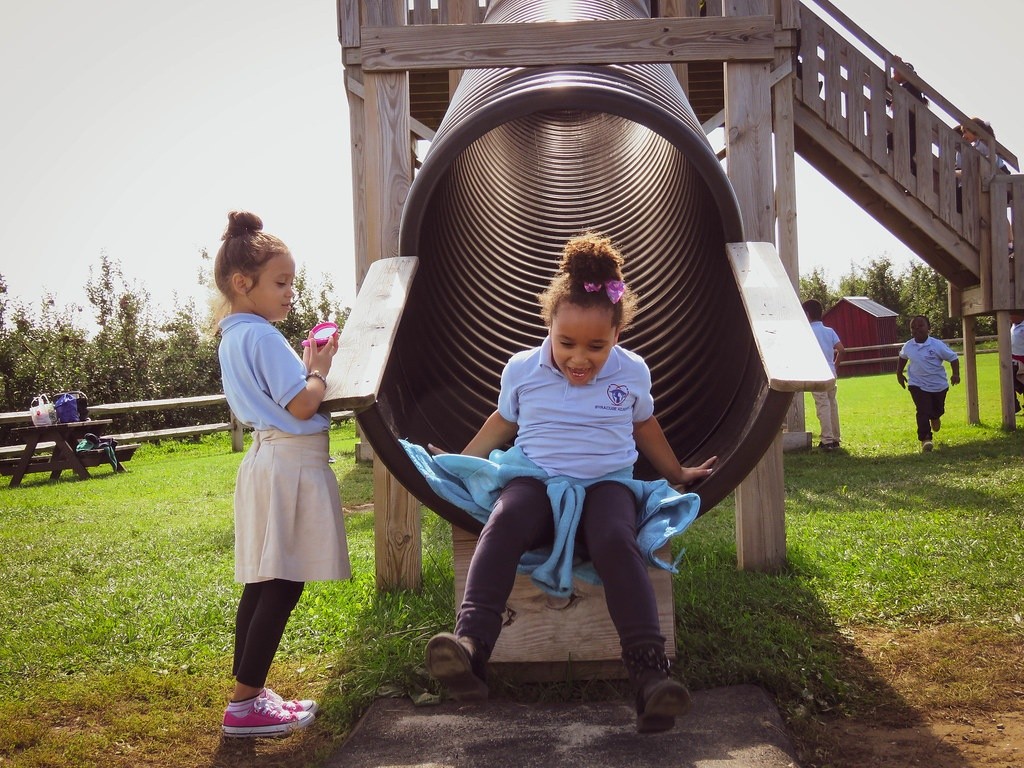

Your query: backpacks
(85, 432), (119, 450)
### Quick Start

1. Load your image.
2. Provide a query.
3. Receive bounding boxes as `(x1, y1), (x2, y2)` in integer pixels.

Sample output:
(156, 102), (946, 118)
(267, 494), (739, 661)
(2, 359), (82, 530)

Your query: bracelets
(306, 371), (329, 391)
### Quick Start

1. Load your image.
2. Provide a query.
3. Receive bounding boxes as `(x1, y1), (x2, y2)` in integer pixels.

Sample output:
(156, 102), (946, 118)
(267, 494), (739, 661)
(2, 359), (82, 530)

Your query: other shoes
(423, 631), (491, 703)
(921, 438), (933, 453)
(634, 670), (692, 733)
(931, 417), (942, 433)
(817, 440), (840, 451)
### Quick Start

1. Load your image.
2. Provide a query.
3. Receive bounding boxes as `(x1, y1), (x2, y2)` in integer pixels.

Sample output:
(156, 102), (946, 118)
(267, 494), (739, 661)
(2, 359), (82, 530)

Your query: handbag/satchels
(28, 394), (58, 426)
(54, 393), (80, 423)
(68, 390), (90, 421)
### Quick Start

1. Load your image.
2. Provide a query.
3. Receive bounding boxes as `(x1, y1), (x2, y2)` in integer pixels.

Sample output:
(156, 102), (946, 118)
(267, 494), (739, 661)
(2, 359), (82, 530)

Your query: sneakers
(259, 688), (318, 718)
(220, 700), (315, 738)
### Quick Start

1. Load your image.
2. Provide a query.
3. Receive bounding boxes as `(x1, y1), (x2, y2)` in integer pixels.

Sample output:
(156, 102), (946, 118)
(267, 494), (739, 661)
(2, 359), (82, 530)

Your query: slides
(353, 1), (797, 554)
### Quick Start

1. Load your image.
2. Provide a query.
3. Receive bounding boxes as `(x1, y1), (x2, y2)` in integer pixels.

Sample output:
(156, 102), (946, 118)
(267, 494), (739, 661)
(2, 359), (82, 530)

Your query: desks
(8, 419), (112, 486)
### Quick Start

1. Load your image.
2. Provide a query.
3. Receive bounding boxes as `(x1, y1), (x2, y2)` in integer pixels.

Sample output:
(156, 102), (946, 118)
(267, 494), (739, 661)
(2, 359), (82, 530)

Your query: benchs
(0, 444), (142, 477)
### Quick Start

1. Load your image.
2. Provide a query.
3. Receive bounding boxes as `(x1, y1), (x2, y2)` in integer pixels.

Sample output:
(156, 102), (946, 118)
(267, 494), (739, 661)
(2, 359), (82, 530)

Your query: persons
(215, 212), (339, 737)
(1009, 314), (1024, 413)
(646, 0), (1016, 255)
(422, 232), (717, 734)
(801, 296), (844, 453)
(897, 316), (961, 455)
